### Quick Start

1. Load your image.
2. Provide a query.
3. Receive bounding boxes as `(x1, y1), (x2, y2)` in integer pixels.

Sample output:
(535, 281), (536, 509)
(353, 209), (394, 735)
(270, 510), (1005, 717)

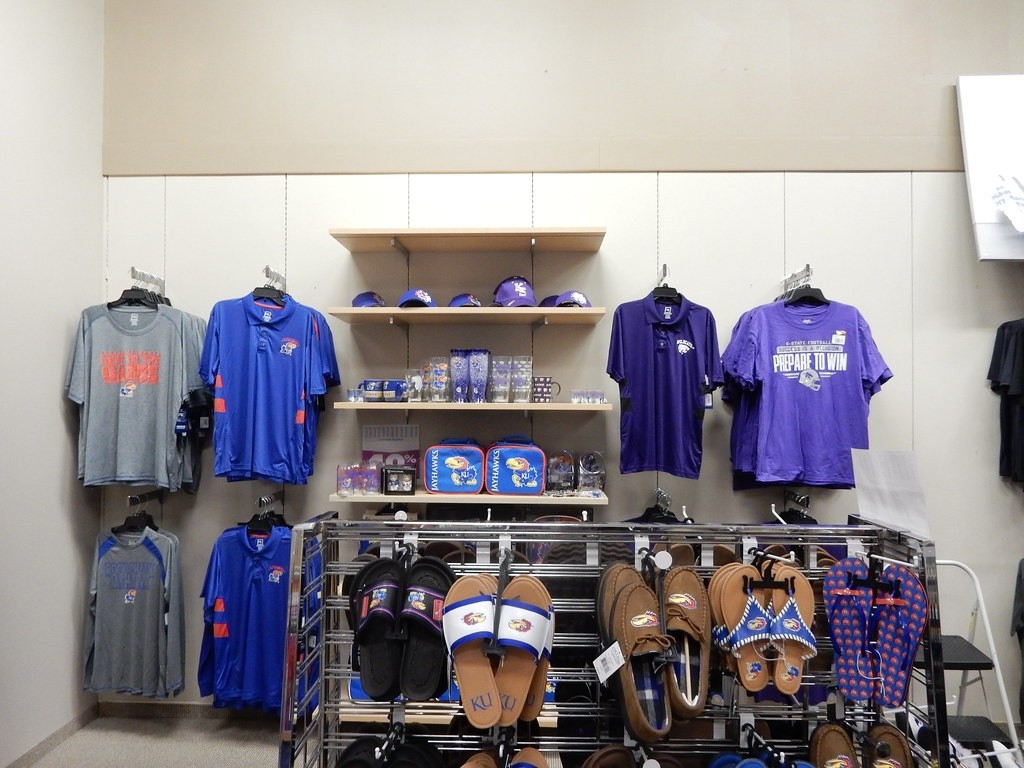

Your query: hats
(351, 290), (386, 308)
(553, 289), (591, 308)
(493, 275), (538, 308)
(396, 289), (437, 308)
(447, 293), (482, 307)
(539, 293), (559, 307)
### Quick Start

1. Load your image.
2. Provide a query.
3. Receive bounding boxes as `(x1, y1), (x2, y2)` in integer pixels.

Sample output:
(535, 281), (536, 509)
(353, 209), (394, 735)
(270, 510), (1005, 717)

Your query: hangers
(618, 487), (694, 525)
(616, 269), (710, 319)
(106, 268), (175, 315)
(756, 271), (857, 319)
(760, 490), (818, 524)
(110, 494), (160, 541)
(224, 491), (295, 539)
(250, 266), (288, 307)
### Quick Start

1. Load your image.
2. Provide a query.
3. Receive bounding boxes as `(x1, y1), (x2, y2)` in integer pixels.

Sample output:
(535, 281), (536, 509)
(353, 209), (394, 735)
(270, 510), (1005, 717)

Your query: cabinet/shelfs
(326, 221), (613, 525)
(275, 512), (950, 768)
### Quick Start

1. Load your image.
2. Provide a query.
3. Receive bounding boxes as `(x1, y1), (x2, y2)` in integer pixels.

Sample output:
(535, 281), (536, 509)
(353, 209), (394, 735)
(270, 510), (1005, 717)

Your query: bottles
(337, 459), (382, 495)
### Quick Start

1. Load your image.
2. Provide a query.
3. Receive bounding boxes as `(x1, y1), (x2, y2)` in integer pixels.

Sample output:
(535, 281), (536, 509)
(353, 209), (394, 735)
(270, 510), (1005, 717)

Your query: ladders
(911, 560), (1022, 768)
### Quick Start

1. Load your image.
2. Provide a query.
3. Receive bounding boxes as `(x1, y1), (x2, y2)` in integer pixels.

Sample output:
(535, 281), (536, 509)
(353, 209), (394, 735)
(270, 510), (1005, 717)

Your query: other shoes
(333, 528), (929, 768)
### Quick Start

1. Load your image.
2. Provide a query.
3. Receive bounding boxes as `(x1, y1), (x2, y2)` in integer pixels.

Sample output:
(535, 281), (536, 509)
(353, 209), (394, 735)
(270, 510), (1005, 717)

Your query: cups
(468, 348), (489, 403)
(571, 391), (608, 403)
(450, 348), (469, 403)
(358, 379), (384, 402)
(347, 387), (364, 403)
(383, 378), (404, 402)
(532, 376), (561, 402)
(491, 355), (512, 402)
(403, 356), (452, 403)
(511, 355), (534, 404)
